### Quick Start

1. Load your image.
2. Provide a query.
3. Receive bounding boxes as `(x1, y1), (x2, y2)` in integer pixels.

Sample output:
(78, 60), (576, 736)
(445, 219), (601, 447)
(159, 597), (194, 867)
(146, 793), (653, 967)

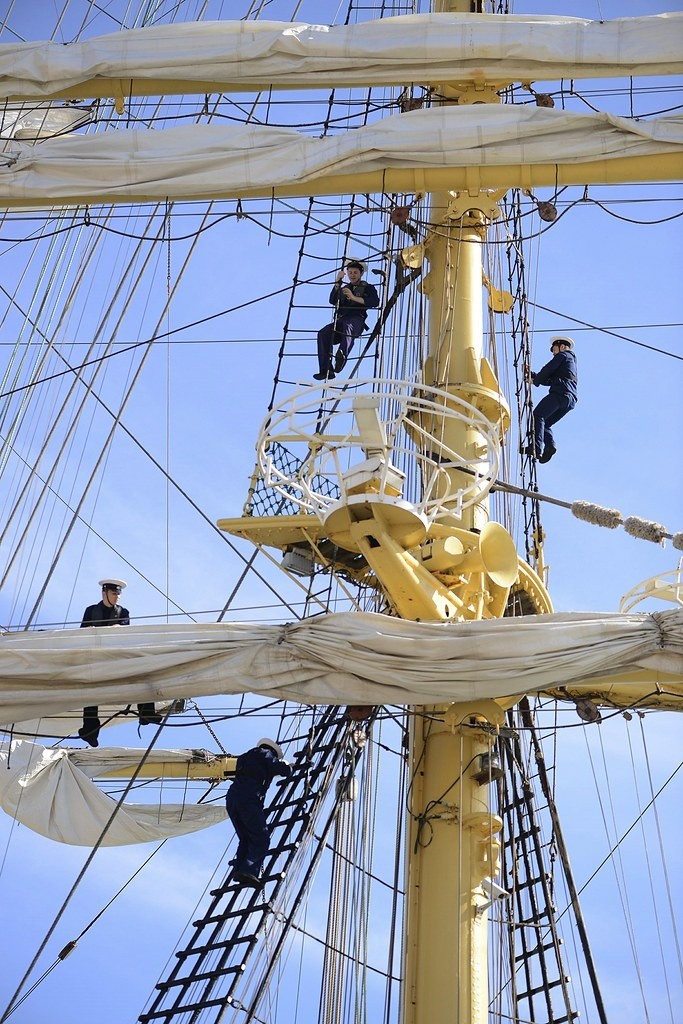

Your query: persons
(519, 336), (577, 464)
(78, 579), (162, 747)
(226, 738), (295, 884)
(313, 261), (380, 380)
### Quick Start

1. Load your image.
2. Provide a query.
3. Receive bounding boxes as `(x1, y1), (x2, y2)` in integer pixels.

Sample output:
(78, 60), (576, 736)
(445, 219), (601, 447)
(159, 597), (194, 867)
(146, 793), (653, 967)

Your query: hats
(549, 335), (575, 352)
(345, 257), (369, 274)
(256, 738), (284, 758)
(98, 579), (127, 595)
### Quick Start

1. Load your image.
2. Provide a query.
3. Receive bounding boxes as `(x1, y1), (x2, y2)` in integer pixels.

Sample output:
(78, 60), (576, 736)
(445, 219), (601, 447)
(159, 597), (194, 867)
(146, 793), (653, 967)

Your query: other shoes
(138, 714), (162, 725)
(313, 371), (336, 380)
(539, 446), (558, 464)
(334, 350), (346, 373)
(234, 872), (260, 884)
(519, 446), (543, 459)
(78, 728), (98, 747)
